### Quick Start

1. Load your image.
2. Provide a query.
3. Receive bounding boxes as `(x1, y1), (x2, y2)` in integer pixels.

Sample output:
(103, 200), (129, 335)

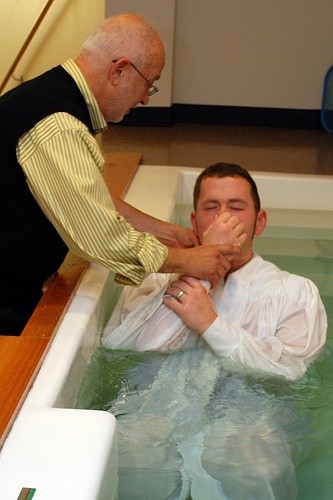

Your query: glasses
(112, 58), (159, 97)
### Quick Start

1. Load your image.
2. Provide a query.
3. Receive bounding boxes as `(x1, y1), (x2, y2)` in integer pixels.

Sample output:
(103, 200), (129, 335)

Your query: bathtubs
(0, 166), (333, 500)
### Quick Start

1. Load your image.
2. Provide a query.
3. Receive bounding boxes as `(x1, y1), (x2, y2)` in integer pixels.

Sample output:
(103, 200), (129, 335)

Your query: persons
(0, 13), (243, 338)
(99, 164), (328, 383)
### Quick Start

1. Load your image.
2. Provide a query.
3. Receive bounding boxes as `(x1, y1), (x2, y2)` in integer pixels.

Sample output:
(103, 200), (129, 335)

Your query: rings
(176, 291), (184, 299)
